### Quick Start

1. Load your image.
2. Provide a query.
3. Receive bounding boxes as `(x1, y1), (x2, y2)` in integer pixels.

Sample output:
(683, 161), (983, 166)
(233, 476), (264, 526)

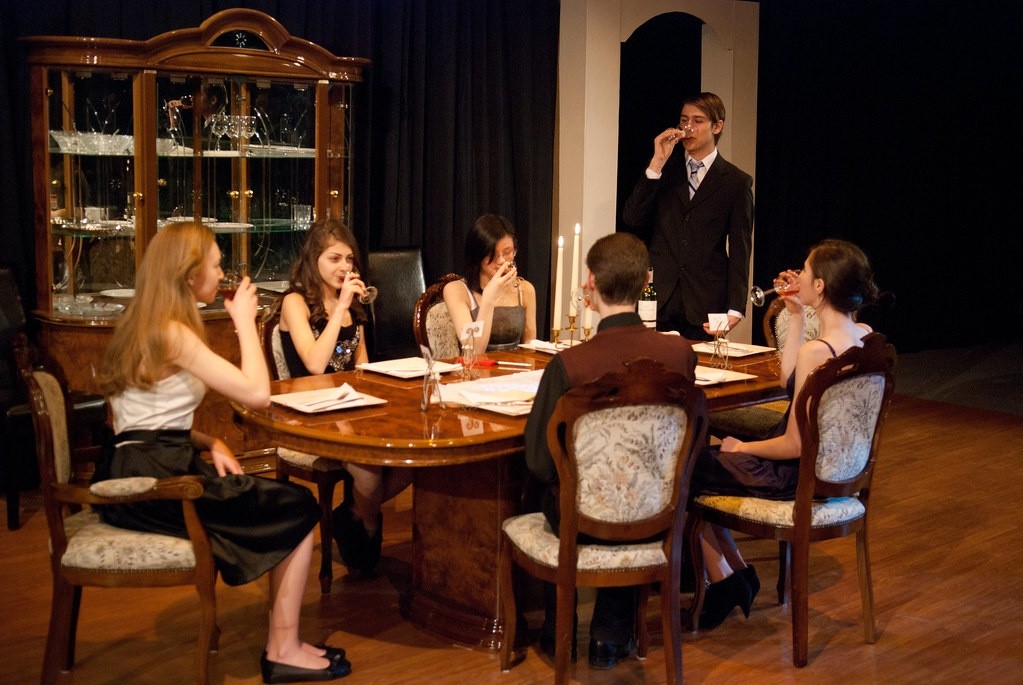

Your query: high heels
(734, 563), (761, 602)
(680, 572), (752, 628)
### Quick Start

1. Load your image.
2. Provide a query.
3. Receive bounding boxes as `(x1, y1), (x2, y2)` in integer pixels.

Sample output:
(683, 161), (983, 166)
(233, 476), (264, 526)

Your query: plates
(203, 223), (254, 229)
(167, 217), (219, 223)
(694, 365), (758, 385)
(58, 302), (125, 316)
(517, 340), (582, 354)
(52, 295), (94, 309)
(196, 302), (207, 308)
(100, 289), (136, 298)
(270, 387), (387, 413)
(354, 357), (462, 378)
(691, 341), (776, 357)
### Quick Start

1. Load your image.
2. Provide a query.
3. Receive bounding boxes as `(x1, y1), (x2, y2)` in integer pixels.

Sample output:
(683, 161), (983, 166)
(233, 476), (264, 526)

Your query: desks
(229, 341), (789, 653)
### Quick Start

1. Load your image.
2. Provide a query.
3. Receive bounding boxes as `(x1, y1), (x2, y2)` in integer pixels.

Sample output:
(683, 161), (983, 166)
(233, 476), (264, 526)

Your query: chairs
(412, 273), (471, 358)
(496, 358), (709, 684)
(0, 268), (113, 533)
(364, 249), (427, 363)
(257, 286), (353, 598)
(710, 297), (857, 438)
(686, 332), (901, 669)
(13, 334), (222, 684)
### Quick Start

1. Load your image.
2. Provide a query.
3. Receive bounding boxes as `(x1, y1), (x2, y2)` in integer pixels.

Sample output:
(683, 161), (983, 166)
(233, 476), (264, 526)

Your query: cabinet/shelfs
(33, 7), (371, 387)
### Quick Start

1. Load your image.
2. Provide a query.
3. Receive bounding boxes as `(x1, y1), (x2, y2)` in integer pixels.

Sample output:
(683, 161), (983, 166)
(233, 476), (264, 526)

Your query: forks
(306, 393), (350, 406)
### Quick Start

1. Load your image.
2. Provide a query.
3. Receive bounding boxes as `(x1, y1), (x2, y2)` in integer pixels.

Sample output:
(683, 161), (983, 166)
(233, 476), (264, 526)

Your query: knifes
(314, 397), (364, 411)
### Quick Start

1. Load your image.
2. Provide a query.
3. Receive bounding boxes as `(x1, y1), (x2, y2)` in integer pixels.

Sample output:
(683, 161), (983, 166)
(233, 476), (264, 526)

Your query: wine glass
(225, 115), (240, 152)
(750, 272), (800, 307)
(498, 257), (526, 293)
(665, 121), (698, 144)
(339, 263), (378, 304)
(217, 270), (278, 323)
(211, 114), (228, 152)
(239, 116), (256, 156)
(164, 95), (192, 111)
(571, 285), (591, 311)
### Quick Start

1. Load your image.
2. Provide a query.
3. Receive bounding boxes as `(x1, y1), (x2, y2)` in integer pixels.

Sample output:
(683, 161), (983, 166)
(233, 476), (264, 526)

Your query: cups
(295, 205), (311, 223)
(100, 208), (108, 222)
(85, 207), (100, 221)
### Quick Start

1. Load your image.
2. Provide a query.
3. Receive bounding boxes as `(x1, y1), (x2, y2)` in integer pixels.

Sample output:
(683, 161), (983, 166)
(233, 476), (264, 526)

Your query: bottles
(638, 267), (657, 331)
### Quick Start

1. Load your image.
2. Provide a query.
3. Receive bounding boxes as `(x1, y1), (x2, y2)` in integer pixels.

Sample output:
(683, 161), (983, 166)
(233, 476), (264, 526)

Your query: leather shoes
(260, 656), (349, 683)
(539, 626), (578, 663)
(262, 644), (345, 660)
(588, 638), (633, 669)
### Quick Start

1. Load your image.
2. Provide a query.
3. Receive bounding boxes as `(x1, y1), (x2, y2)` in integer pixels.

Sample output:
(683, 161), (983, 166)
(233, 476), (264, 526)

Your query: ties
(688, 159), (705, 200)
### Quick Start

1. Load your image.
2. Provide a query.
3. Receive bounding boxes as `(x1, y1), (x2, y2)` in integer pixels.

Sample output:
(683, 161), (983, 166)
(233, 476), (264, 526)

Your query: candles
(569, 222), (581, 316)
(553, 236), (564, 330)
(584, 306), (592, 329)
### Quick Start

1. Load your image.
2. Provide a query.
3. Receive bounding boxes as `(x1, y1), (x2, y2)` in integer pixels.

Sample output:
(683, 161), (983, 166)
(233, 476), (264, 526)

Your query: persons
(442, 214), (537, 356)
(619, 90), (753, 340)
(279, 217), (416, 576)
(523, 235), (698, 671)
(681, 240), (881, 630)
(95, 221), (352, 683)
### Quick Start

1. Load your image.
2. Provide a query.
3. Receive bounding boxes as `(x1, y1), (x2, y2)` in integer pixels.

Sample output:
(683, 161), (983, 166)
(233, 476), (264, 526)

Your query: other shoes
(329, 504), (383, 570)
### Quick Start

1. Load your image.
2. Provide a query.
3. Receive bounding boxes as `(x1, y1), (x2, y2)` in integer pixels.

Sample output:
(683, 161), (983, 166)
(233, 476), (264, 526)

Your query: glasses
(485, 248), (518, 261)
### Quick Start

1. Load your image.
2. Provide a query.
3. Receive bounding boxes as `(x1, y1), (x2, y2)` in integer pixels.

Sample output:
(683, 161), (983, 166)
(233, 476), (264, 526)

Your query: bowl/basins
(77, 134), (174, 155)
(49, 130), (101, 152)
